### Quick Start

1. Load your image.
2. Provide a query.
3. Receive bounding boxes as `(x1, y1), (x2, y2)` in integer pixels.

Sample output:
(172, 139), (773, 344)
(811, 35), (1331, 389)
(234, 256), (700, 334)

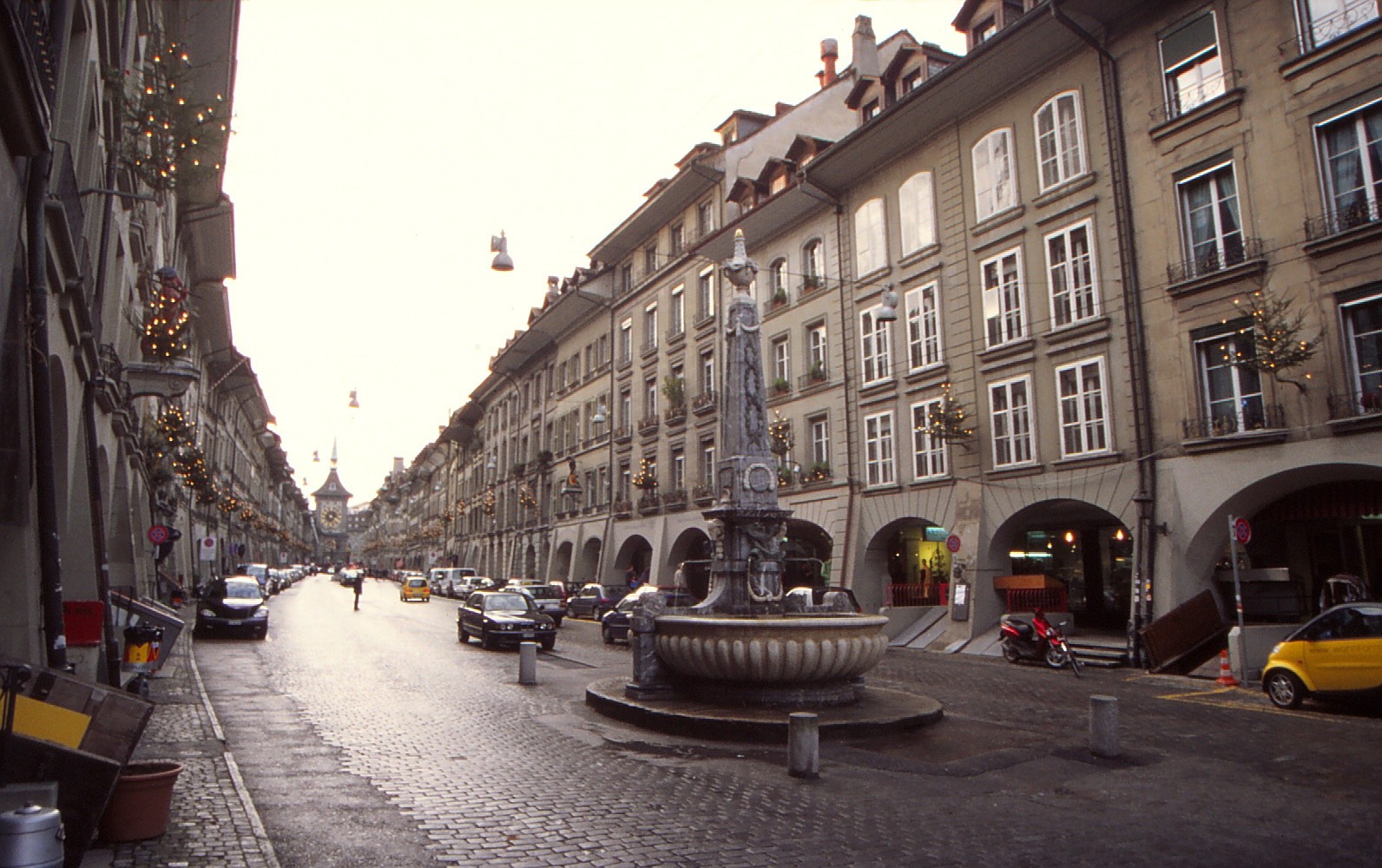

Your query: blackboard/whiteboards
(951, 577), (970, 622)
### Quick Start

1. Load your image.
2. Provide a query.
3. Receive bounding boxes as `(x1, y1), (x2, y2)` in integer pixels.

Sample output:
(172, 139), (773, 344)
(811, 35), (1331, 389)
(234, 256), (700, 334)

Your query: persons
(674, 561), (686, 586)
(625, 563), (637, 593)
(353, 572), (362, 611)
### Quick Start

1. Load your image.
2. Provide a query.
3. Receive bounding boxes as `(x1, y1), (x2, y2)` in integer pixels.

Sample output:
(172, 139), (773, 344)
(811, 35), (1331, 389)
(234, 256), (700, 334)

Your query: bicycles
(1044, 620), (1085, 679)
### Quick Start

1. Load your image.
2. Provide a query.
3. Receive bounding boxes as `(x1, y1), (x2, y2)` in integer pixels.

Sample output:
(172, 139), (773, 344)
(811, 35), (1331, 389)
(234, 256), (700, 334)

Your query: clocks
(320, 506), (343, 529)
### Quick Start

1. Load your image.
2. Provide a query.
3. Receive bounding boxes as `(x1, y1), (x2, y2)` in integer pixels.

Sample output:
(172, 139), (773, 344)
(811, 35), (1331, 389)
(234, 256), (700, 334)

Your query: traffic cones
(1216, 650), (1241, 687)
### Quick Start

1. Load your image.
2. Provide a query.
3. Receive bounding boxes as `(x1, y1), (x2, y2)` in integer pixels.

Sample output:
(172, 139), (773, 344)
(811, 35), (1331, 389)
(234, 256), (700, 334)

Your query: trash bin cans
(121, 626), (166, 672)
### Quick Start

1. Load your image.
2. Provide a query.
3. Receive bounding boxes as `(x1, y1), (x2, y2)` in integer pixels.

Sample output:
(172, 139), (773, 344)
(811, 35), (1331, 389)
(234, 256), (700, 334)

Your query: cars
(399, 576), (430, 603)
(327, 567), (365, 586)
(452, 576), (486, 601)
(601, 584), (698, 644)
(194, 574), (270, 639)
(1262, 602), (1382, 708)
(391, 569), (423, 584)
(503, 584), (568, 629)
(474, 578), (509, 592)
(508, 578), (543, 586)
(549, 581), (584, 600)
(566, 583), (633, 621)
(268, 563), (324, 594)
(456, 591), (557, 650)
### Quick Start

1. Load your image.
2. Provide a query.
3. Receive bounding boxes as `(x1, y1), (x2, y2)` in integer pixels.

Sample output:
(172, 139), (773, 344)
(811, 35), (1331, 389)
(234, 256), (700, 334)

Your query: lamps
(877, 286), (898, 321)
(488, 457), (497, 469)
(594, 404), (607, 423)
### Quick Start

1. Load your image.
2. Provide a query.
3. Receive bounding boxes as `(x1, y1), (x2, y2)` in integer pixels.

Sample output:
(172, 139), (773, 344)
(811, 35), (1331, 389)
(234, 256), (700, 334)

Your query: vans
(429, 566), (477, 598)
(236, 563), (271, 599)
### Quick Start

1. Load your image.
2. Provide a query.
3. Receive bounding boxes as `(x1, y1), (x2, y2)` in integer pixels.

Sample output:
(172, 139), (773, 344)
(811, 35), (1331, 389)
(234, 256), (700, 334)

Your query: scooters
(997, 608), (1068, 670)
